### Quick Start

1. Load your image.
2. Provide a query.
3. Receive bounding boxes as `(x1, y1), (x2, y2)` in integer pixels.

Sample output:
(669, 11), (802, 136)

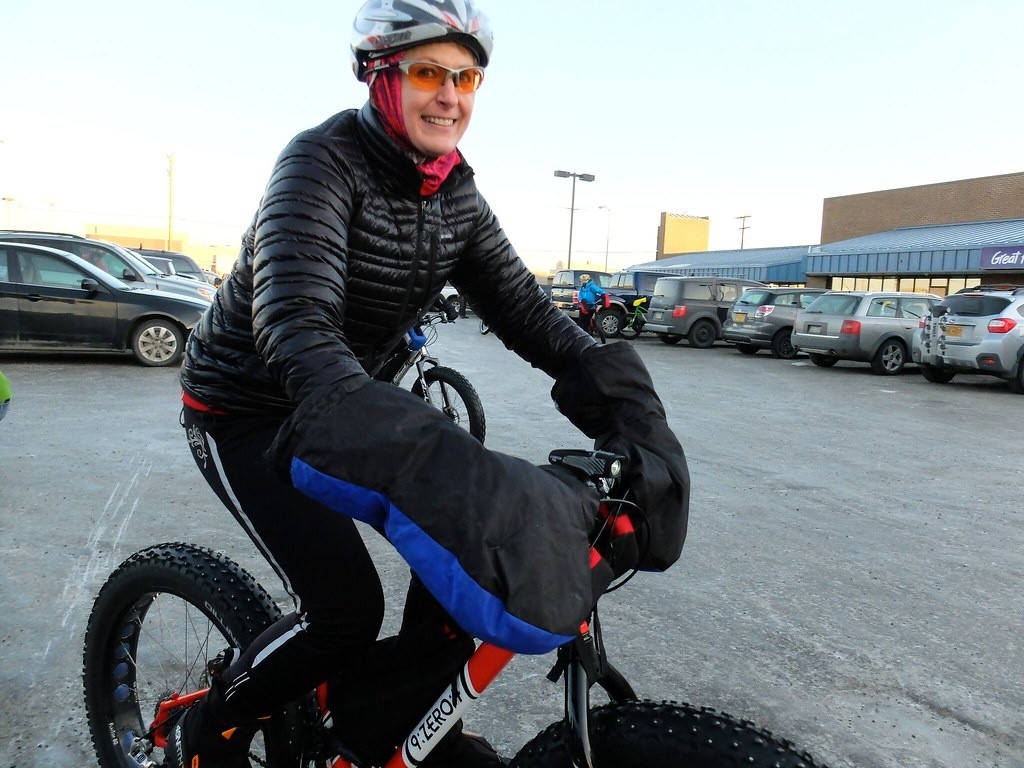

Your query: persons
(162, 0), (691, 768)
(579, 273), (607, 345)
(432, 294), (469, 324)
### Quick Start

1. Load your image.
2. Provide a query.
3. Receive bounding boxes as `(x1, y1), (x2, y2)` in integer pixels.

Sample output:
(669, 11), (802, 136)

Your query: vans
(643, 277), (768, 348)
(912, 283), (1024, 389)
(790, 289), (942, 373)
(722, 287), (833, 359)
(549, 270), (612, 322)
(721, 287), (834, 359)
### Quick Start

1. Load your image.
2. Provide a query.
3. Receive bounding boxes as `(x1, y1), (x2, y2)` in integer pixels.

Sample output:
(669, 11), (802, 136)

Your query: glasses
(362, 61), (485, 94)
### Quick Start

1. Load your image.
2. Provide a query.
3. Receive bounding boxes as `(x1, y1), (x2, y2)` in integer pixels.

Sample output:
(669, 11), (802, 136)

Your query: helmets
(579, 274), (590, 282)
(350, 0), (492, 82)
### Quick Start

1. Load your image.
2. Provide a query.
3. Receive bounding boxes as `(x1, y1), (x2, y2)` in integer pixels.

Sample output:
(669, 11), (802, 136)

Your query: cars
(0, 228), (223, 366)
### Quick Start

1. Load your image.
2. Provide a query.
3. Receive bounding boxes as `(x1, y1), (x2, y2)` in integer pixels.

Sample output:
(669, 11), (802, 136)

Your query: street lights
(552, 166), (596, 273)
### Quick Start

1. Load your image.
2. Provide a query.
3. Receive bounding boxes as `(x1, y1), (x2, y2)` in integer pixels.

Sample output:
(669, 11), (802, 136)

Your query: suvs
(584, 272), (658, 339)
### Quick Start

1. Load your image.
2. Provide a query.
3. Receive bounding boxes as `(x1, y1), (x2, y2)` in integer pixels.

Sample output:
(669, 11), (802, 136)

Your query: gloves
(602, 293), (611, 307)
(578, 301), (589, 314)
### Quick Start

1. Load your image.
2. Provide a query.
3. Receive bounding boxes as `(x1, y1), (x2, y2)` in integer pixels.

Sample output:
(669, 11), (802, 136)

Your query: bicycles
(81, 448), (834, 768)
(617, 297), (658, 339)
(375, 299), (486, 447)
